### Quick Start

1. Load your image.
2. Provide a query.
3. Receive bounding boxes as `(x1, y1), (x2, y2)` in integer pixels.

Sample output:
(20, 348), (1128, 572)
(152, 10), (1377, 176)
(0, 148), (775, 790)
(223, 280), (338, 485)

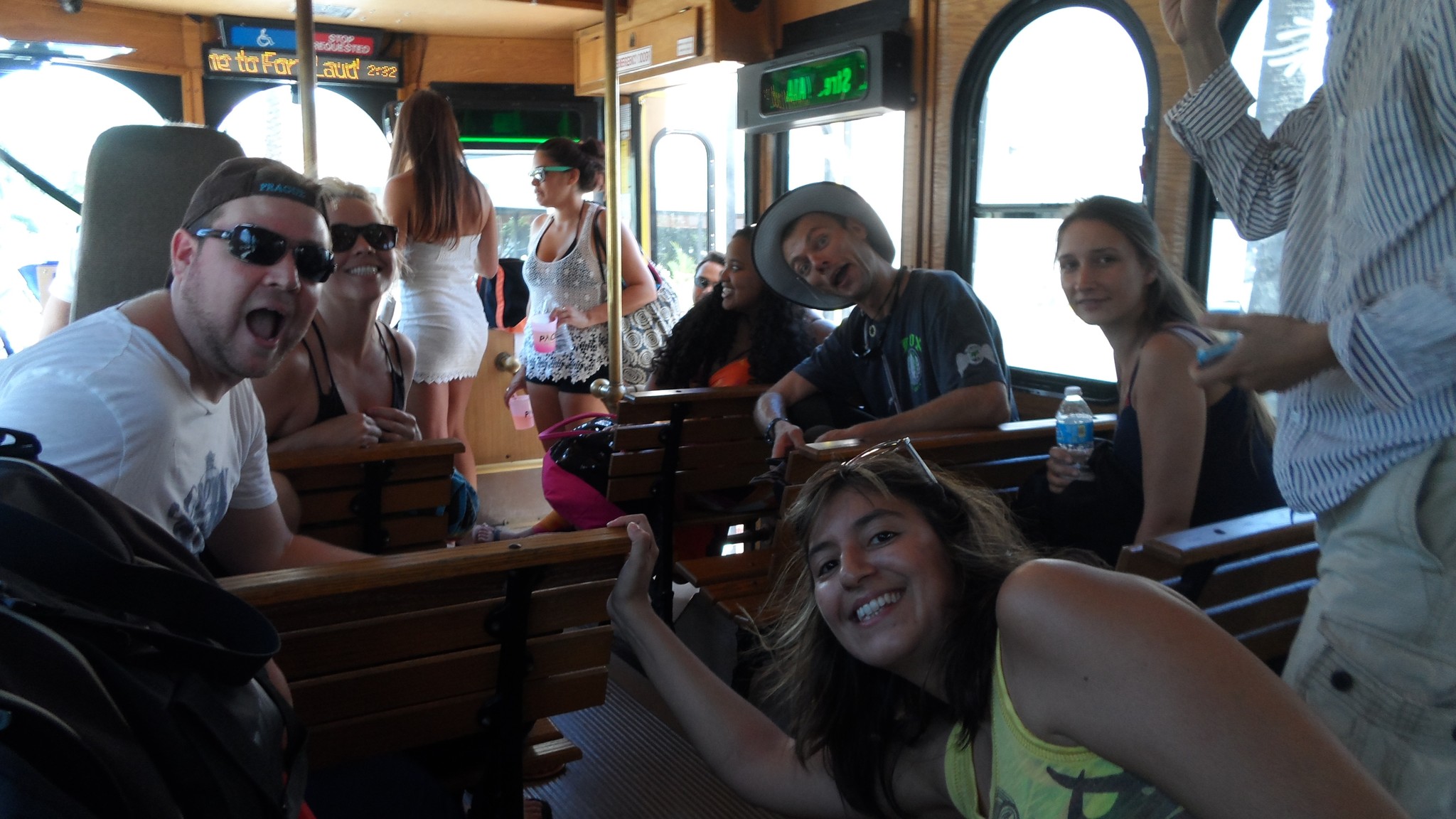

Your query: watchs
(760, 417), (792, 451)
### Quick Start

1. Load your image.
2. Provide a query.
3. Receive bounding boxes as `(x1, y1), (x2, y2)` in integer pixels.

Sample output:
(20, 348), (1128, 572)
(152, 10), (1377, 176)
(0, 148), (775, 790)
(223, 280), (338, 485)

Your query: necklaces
(859, 265), (907, 356)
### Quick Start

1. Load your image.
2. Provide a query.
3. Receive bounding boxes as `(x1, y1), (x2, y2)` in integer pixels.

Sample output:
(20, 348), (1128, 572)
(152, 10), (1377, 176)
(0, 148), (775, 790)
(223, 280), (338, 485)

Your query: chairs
(70, 125), (247, 327)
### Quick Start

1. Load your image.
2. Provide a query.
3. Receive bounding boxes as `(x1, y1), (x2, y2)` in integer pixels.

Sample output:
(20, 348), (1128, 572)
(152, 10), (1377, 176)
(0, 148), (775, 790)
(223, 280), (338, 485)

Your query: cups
(508, 394), (535, 430)
(533, 314), (558, 352)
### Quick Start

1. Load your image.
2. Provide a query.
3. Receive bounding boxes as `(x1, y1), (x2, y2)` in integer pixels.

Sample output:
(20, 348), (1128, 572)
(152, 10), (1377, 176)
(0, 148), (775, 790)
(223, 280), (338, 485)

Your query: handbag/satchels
(600, 261), (679, 386)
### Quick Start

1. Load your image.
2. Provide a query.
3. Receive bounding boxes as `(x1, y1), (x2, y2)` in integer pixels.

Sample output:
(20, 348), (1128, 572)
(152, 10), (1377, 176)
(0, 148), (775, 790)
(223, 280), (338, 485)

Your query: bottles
(542, 295), (575, 350)
(1055, 384), (1104, 480)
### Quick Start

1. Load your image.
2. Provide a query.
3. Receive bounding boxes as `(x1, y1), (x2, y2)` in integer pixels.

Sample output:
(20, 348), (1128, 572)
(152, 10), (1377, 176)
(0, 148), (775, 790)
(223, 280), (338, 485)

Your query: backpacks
(0, 428), (311, 819)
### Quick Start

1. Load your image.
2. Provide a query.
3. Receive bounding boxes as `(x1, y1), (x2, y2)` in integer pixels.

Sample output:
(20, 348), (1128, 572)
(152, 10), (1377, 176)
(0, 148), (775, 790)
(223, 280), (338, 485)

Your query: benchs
(673, 414), (1120, 701)
(261, 437), (463, 554)
(212, 521), (632, 818)
(1113, 503), (1317, 679)
(609, 388), (789, 634)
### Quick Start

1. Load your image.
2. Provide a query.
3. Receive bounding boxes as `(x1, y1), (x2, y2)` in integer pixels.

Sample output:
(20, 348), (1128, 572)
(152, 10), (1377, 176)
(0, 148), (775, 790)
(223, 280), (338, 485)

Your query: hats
(164, 156), (329, 285)
(752, 183), (897, 311)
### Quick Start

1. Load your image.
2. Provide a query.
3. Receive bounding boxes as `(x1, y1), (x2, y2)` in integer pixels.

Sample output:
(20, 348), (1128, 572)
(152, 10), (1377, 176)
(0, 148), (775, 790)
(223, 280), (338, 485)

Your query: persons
(469, 221), (836, 548)
(1148, 0), (1456, 818)
(31, 226), (82, 346)
(0, 155), (379, 574)
(1034, 191), (1288, 573)
(502, 137), (659, 454)
(690, 251), (726, 305)
(247, 176), (425, 460)
(381, 88), (502, 546)
(605, 436), (1411, 819)
(751, 181), (1022, 503)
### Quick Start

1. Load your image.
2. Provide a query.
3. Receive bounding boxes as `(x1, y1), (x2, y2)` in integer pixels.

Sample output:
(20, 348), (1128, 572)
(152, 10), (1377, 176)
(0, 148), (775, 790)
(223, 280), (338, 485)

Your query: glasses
(528, 166), (574, 183)
(191, 223), (336, 284)
(843, 433), (939, 489)
(330, 223), (400, 252)
(693, 276), (720, 290)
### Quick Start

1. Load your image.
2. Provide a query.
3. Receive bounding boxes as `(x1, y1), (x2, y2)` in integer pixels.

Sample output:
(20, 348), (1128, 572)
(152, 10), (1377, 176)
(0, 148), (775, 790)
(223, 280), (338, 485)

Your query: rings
(506, 386), (510, 392)
(560, 304), (566, 311)
